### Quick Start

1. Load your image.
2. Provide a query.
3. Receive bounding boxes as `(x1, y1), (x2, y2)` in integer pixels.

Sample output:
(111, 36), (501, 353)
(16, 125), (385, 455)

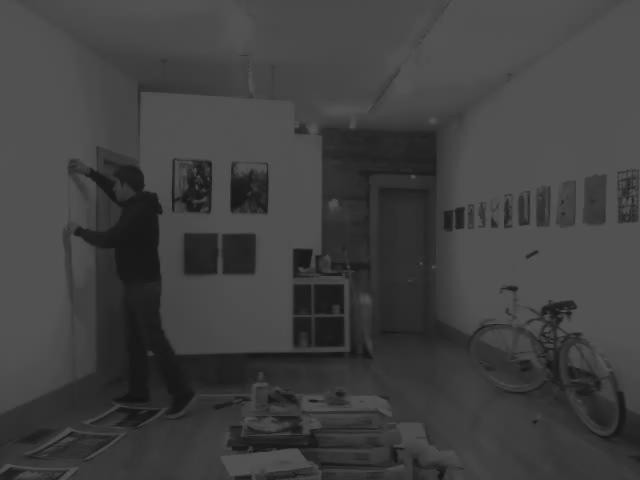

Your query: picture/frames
(170, 158), (213, 215)
(229, 159), (270, 216)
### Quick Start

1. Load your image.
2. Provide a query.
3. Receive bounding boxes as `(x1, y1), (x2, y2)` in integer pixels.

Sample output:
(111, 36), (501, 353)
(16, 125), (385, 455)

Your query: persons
(68, 158), (196, 419)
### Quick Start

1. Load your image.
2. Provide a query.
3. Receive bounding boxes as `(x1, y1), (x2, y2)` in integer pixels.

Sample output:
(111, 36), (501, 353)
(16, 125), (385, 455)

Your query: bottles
(297, 121), (308, 134)
(249, 371), (269, 414)
(298, 330), (309, 347)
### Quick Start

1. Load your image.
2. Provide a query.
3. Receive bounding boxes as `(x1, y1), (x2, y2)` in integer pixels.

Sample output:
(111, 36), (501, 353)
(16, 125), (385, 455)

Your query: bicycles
(462, 249), (628, 443)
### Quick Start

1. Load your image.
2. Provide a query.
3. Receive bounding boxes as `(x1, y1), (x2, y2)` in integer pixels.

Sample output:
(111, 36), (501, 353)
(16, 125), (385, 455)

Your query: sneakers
(165, 392), (195, 419)
(112, 393), (149, 406)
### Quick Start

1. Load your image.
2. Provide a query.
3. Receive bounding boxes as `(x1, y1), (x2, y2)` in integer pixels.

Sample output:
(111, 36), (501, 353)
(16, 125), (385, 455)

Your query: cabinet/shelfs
(293, 276), (351, 355)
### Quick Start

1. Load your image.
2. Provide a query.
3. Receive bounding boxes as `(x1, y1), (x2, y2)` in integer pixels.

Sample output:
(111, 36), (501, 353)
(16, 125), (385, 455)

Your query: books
(221, 396), (321, 479)
